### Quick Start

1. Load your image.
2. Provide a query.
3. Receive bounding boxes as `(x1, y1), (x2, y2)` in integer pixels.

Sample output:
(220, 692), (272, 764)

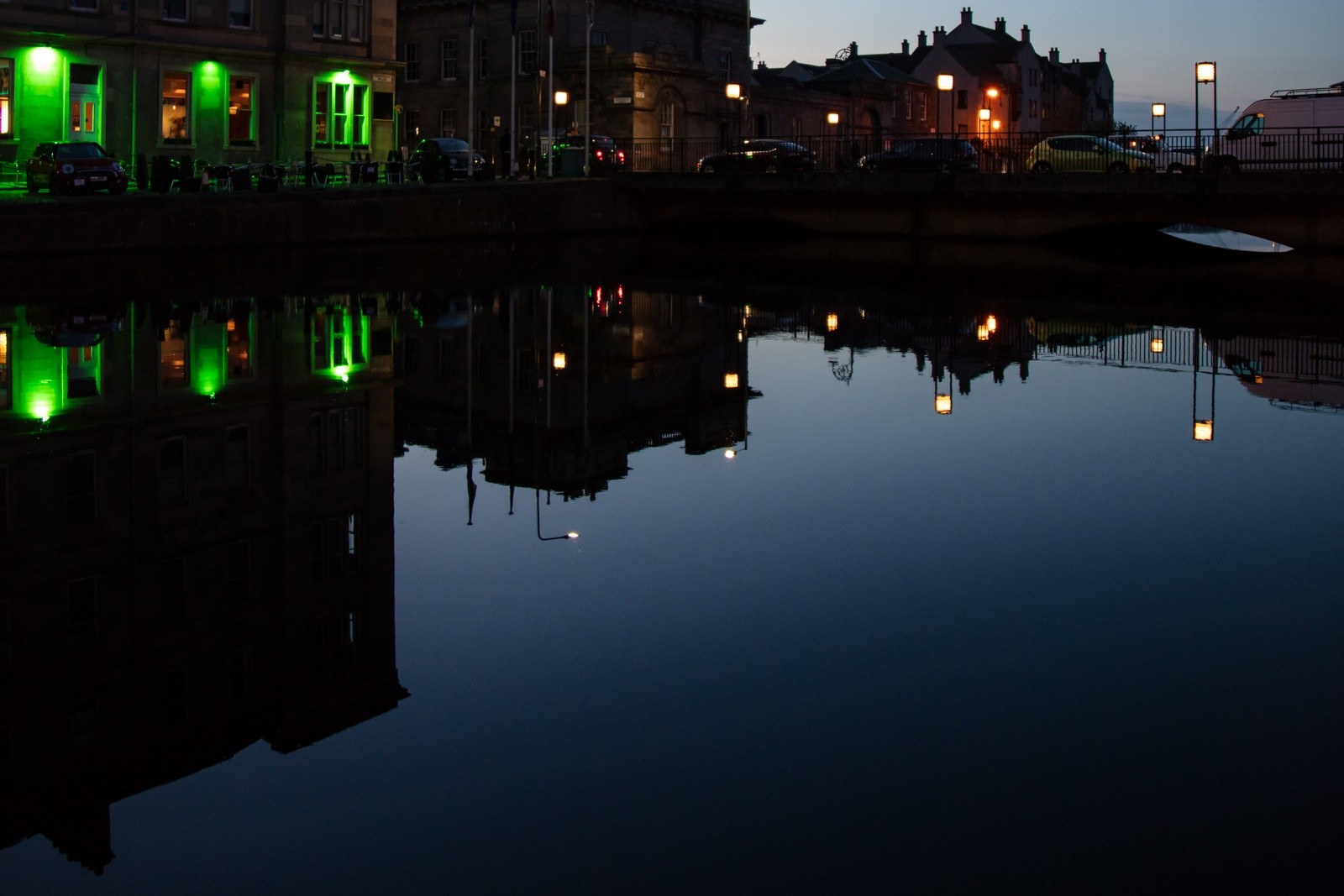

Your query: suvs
(1105, 134), (1203, 175)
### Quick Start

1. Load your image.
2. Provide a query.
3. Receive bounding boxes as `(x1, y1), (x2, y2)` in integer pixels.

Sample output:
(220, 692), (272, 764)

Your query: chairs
(194, 159), (403, 194)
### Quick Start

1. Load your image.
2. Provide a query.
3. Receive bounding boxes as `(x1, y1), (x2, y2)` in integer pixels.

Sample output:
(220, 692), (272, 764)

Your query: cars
(551, 133), (625, 170)
(26, 140), (129, 197)
(856, 136), (981, 175)
(697, 138), (820, 179)
(405, 136), (490, 184)
(1025, 134), (1156, 176)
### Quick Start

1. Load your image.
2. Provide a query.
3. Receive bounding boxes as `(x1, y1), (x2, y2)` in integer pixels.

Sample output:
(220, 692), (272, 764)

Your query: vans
(1202, 81), (1344, 172)
